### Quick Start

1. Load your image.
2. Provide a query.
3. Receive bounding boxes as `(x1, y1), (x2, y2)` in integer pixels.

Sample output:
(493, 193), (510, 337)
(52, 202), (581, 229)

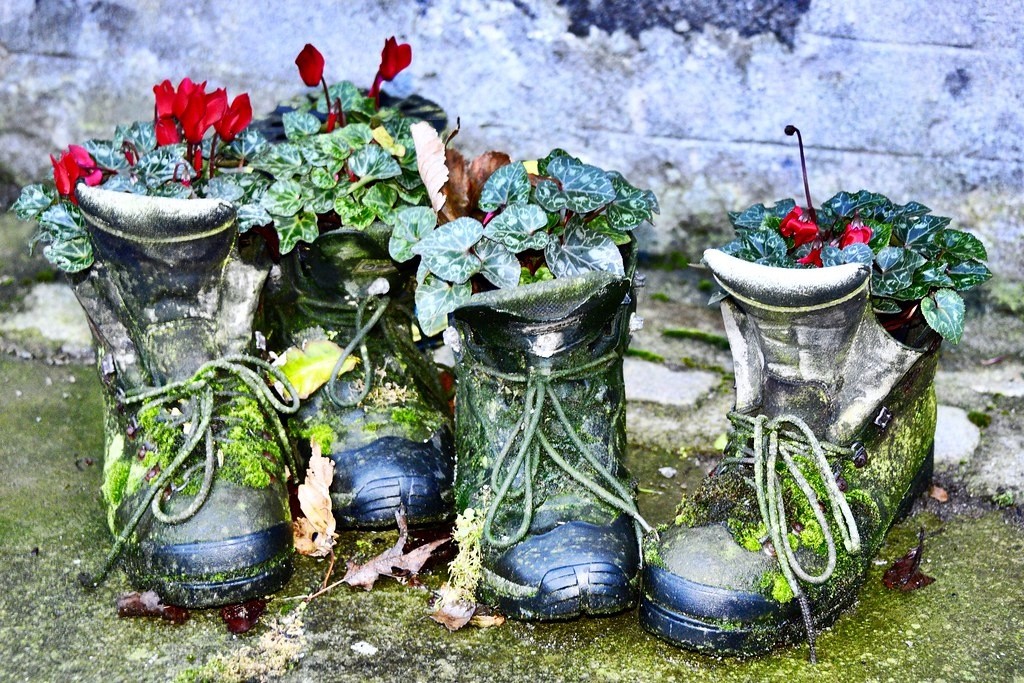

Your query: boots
(636, 249), (945, 664)
(440, 228), (660, 621)
(242, 226), (457, 527)
(35, 139), (313, 606)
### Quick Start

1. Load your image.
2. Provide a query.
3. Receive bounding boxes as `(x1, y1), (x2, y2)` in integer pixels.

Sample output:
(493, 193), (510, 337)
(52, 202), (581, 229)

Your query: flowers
(23, 78), (251, 224)
(727, 186), (991, 343)
(246, 32), (443, 227)
(411, 147), (663, 336)
